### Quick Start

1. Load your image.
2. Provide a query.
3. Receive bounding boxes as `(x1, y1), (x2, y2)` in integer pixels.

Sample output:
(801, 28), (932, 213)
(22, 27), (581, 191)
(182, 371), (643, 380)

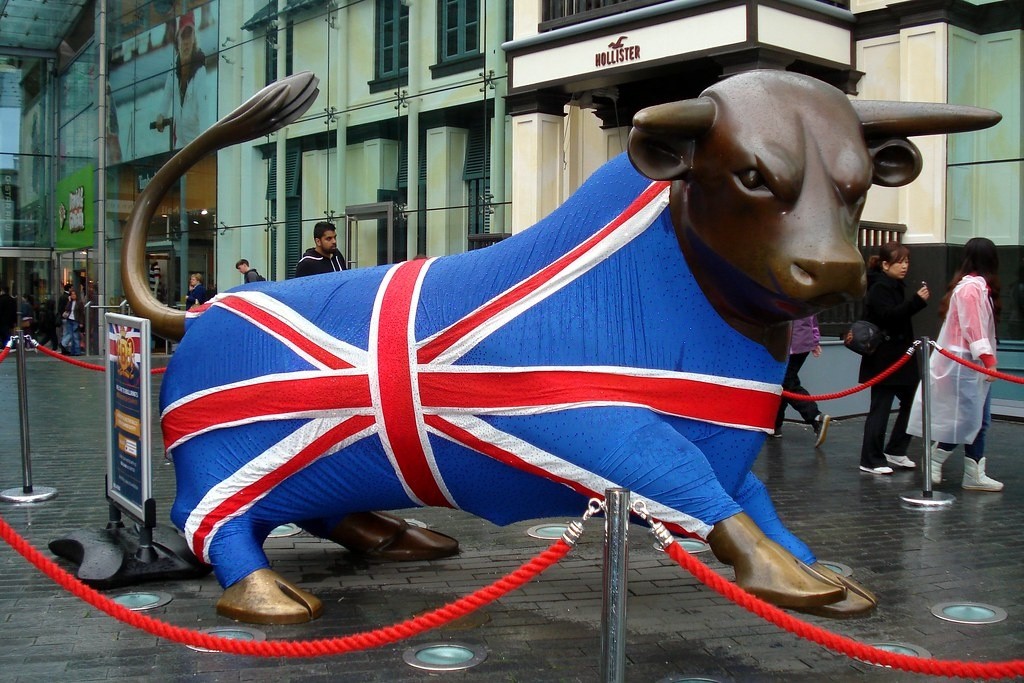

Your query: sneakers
(883, 452), (916, 469)
(859, 465), (892, 474)
(813, 415), (831, 448)
(773, 429), (783, 438)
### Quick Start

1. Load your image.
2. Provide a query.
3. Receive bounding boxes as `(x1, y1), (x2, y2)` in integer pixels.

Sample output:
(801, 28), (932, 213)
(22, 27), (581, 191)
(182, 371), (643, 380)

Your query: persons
(844, 241), (931, 476)
(149, 255), (162, 303)
(293, 220), (347, 278)
(905, 236), (1006, 492)
(19, 293), (37, 352)
(0, 285), (18, 351)
(154, 9), (210, 149)
(105, 79), (125, 168)
(64, 291), (84, 357)
(55, 283), (74, 354)
(184, 272), (208, 311)
(234, 258), (266, 285)
(773, 311), (832, 448)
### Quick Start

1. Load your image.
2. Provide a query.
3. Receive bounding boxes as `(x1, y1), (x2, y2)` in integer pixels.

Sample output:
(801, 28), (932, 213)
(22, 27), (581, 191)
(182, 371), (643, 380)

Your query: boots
(961, 455), (1004, 491)
(922, 440), (951, 484)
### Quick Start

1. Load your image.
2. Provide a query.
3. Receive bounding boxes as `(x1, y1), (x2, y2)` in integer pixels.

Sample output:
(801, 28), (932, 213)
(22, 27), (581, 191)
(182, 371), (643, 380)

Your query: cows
(122, 69), (1002, 626)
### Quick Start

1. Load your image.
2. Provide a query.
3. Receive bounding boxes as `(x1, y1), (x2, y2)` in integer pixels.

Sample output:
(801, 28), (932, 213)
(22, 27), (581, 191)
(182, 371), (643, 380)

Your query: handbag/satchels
(845, 319), (885, 354)
